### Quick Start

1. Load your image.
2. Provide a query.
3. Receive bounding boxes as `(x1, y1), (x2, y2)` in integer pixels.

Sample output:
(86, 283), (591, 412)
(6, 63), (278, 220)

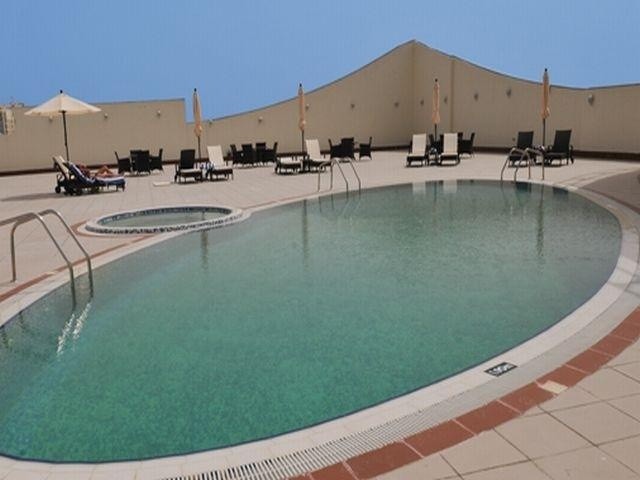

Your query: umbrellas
(540, 68), (552, 146)
(431, 76), (440, 142)
(23, 89), (102, 159)
(193, 87), (203, 163)
(297, 82), (307, 159)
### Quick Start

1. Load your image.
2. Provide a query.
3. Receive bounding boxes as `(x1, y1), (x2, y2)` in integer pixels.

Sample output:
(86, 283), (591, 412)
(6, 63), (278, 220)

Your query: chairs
(173, 147), (234, 186)
(229, 140), (280, 168)
(275, 134), (374, 177)
(407, 132), (475, 170)
(52, 155), (124, 199)
(507, 129), (574, 170)
(113, 147), (167, 175)
